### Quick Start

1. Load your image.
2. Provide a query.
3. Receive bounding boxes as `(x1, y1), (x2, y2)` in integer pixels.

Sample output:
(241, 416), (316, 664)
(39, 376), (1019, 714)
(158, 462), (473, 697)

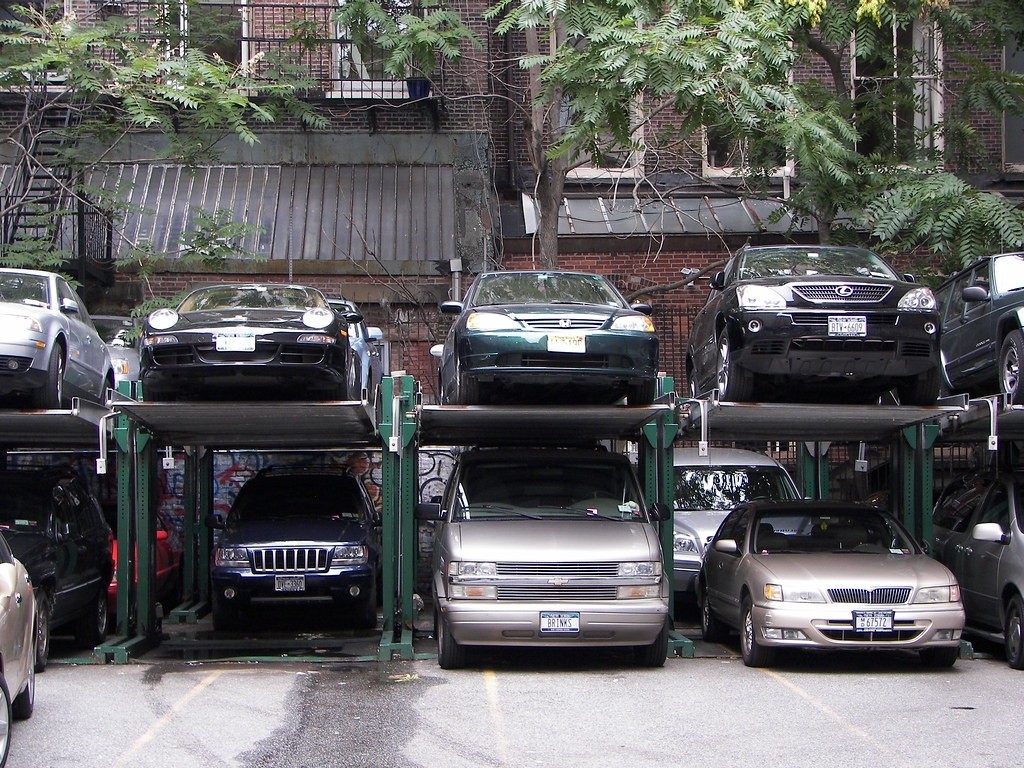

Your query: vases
(407, 76), (430, 99)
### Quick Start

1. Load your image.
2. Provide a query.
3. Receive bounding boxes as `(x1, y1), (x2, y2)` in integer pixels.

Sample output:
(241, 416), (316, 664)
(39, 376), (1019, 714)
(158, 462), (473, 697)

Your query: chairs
(755, 523), (867, 550)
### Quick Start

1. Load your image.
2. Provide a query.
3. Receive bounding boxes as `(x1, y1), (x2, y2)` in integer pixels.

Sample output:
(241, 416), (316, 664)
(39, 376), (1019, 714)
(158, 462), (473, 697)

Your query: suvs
(204, 458), (384, 638)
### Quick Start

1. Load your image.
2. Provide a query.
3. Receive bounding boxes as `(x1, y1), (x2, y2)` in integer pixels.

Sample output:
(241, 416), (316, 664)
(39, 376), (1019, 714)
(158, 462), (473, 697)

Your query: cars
(429, 269), (659, 407)
(0, 268), (115, 410)
(139, 281), (364, 402)
(0, 462), (114, 673)
(0, 532), (38, 768)
(698, 496), (965, 672)
(88, 314), (142, 390)
(108, 510), (182, 627)
(685, 244), (940, 406)
(326, 298), (383, 406)
(934, 251), (1024, 405)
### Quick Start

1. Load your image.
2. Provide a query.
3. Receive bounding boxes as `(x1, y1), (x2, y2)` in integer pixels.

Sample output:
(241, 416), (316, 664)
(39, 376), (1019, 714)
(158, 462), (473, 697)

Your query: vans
(414, 445), (670, 669)
(672, 447), (802, 626)
(930, 470), (1024, 672)
